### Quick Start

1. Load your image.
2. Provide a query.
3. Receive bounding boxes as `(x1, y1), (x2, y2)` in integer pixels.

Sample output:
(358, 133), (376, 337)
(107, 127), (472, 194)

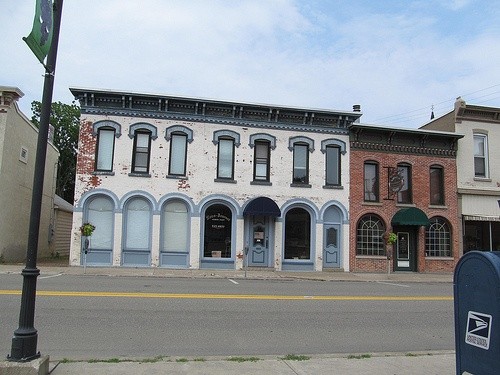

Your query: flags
(23, 0), (53, 63)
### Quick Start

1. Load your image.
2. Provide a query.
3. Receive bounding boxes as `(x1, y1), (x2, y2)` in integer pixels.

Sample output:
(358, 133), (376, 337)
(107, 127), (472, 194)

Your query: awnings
(391, 206), (431, 227)
(240, 197), (282, 216)
